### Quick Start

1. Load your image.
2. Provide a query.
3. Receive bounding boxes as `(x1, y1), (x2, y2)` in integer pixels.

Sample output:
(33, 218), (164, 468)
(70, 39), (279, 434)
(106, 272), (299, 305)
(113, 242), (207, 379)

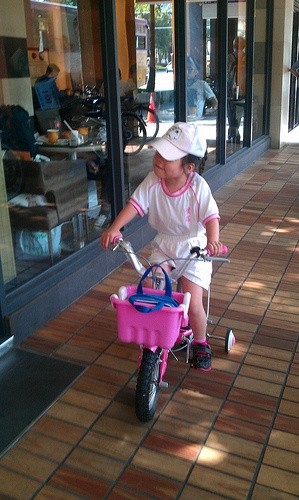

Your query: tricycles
(106, 234), (238, 421)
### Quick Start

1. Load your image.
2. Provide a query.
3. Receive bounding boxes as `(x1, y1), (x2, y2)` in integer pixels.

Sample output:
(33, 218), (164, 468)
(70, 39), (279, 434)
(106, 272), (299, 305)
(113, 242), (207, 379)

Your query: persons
(35, 64), (81, 117)
(290, 61), (299, 78)
(186, 56), (219, 174)
(99, 122), (224, 373)
(228, 36), (248, 144)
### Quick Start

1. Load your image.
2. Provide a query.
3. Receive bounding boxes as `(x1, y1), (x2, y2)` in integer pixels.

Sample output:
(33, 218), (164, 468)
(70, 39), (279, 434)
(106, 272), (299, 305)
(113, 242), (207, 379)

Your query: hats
(150, 122), (207, 161)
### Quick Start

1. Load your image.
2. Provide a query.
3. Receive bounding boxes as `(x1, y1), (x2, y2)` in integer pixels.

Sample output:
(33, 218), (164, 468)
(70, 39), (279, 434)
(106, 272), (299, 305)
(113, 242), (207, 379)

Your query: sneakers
(192, 343), (213, 371)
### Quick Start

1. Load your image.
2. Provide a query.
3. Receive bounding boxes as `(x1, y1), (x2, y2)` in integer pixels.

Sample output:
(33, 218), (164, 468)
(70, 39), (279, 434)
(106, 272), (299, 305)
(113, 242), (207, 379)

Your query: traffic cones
(146, 93), (162, 123)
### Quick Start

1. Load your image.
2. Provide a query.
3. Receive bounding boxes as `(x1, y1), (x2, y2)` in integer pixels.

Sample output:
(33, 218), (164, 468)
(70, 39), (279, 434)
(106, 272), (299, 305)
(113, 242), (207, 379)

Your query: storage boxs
(35, 75), (61, 110)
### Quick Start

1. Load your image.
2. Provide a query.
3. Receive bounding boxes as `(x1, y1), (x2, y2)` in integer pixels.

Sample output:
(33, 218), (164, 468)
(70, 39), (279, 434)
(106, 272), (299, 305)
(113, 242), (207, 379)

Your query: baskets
(109, 285), (192, 349)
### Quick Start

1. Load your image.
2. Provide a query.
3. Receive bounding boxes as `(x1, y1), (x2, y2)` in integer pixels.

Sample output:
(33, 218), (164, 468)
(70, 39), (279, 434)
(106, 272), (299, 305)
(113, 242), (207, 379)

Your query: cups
(79, 127), (89, 143)
(65, 130), (70, 137)
(47, 129), (60, 144)
(69, 130), (78, 145)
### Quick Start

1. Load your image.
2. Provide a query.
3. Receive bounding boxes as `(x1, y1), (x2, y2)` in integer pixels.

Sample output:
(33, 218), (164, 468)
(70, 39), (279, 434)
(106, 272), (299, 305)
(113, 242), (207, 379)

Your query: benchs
(4, 158), (90, 261)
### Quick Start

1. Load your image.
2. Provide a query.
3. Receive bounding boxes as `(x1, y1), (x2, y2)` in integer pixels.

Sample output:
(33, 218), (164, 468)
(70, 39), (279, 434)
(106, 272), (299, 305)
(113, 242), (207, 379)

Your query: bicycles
(84, 85), (160, 156)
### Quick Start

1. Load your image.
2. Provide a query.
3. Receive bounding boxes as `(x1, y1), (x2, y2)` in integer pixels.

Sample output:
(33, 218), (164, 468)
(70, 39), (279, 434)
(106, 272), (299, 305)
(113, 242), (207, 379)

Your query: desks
(38, 142), (105, 248)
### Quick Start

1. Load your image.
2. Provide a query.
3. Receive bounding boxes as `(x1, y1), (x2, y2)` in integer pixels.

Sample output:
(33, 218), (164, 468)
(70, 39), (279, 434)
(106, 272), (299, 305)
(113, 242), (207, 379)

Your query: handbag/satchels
(128, 264), (179, 313)
